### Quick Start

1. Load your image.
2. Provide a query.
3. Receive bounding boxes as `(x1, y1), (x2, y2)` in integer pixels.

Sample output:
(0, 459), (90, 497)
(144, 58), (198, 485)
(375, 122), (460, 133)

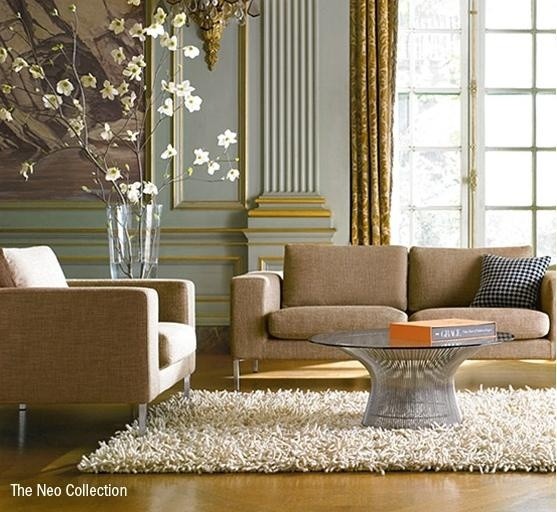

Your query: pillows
(471, 250), (551, 310)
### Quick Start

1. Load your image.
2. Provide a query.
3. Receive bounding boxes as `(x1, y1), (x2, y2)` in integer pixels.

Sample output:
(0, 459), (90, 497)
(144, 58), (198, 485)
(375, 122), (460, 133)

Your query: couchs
(228, 244), (557, 391)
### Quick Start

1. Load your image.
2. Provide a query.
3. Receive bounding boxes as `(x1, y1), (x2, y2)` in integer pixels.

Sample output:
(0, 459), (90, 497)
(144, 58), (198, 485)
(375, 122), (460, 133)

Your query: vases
(104, 203), (162, 280)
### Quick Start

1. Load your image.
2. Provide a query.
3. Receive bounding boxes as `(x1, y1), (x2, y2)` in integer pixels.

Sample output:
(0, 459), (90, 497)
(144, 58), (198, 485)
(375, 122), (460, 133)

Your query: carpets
(74, 385), (557, 473)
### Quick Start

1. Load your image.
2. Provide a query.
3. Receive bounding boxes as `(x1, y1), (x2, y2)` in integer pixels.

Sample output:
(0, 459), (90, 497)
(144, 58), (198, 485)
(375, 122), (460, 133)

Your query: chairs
(0, 244), (197, 444)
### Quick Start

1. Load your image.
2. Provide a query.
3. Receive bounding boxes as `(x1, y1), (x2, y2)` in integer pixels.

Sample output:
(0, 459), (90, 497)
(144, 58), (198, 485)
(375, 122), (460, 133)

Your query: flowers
(0, 0), (243, 279)
